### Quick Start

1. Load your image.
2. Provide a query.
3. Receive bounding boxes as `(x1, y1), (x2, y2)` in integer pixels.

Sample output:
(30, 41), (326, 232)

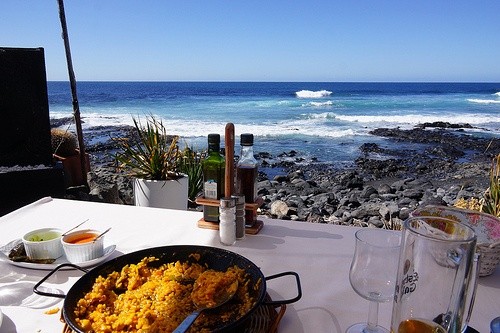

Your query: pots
(32, 244), (303, 333)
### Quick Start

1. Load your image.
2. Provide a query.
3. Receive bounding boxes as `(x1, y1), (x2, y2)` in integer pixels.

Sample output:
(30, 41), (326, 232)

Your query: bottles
(234, 133), (258, 203)
(218, 197), (239, 246)
(200, 133), (226, 223)
(231, 193), (246, 239)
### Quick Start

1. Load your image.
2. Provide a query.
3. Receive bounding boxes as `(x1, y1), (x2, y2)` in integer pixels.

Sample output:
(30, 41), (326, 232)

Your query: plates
(0, 237), (117, 270)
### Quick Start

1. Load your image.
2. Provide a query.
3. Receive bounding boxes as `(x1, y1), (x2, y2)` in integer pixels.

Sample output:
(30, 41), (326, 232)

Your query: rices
(44, 253), (260, 333)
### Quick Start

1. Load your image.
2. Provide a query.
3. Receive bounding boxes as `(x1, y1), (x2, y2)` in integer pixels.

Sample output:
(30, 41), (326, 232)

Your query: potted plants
(119, 113), (196, 211)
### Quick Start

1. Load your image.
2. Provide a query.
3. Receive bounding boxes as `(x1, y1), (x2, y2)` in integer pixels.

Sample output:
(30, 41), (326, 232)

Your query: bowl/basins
(61, 229), (105, 264)
(22, 227), (66, 261)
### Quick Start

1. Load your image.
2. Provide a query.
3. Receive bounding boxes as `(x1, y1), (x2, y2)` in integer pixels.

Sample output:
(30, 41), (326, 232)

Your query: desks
(0, 195), (500, 333)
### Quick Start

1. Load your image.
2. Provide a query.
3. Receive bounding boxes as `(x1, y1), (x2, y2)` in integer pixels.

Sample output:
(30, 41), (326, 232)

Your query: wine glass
(345, 226), (417, 333)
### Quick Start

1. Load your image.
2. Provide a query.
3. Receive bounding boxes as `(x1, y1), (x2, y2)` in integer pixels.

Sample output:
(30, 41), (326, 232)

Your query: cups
(389, 215), (477, 333)
(397, 318), (447, 333)
